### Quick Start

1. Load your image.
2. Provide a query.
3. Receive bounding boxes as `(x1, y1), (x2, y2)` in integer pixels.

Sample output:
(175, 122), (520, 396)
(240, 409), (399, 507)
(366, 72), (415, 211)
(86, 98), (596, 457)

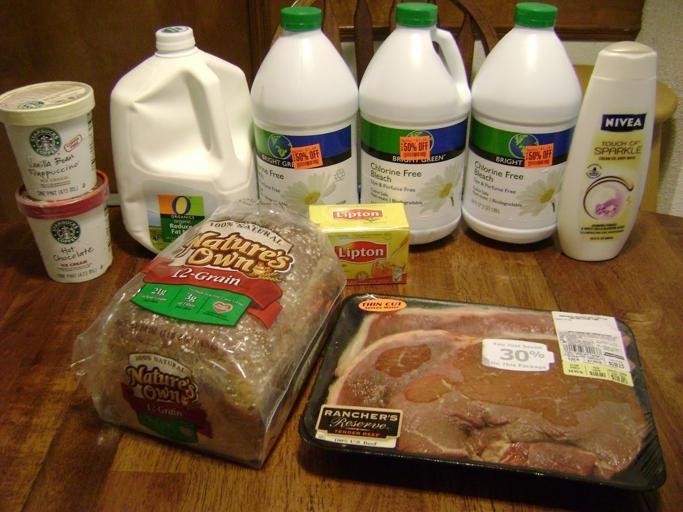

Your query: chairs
(264, 1), (504, 75)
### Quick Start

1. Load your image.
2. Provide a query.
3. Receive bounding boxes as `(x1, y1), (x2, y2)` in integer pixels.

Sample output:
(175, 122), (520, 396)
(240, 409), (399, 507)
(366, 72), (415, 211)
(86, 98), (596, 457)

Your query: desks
(0, 199), (681, 512)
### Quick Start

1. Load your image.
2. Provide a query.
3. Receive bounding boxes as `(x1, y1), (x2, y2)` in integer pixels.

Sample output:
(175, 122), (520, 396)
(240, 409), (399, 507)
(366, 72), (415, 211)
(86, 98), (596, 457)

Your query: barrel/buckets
(108, 24), (257, 256)
(461, 1), (583, 246)
(358, 0), (471, 247)
(251, 6), (360, 222)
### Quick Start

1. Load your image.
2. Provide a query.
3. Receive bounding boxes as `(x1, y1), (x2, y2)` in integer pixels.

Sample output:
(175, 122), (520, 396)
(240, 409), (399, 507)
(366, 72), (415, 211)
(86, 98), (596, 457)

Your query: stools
(566, 61), (678, 211)
